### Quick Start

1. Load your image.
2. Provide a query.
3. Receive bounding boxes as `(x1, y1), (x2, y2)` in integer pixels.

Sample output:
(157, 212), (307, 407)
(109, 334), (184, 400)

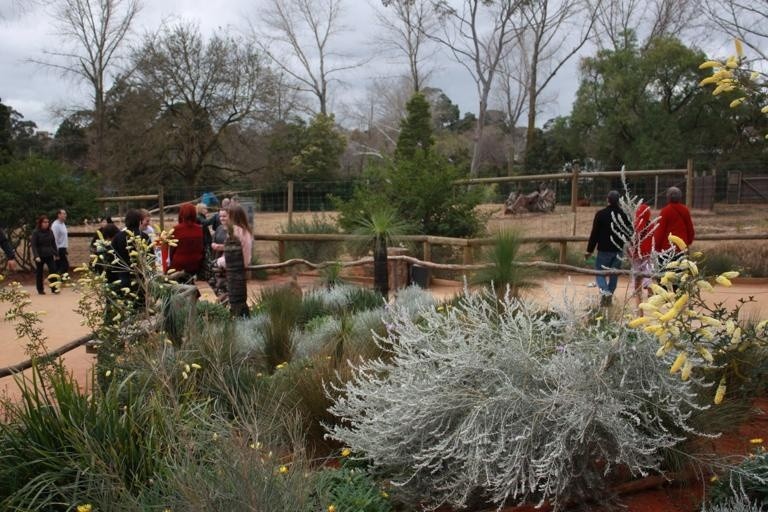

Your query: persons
(30, 214), (61, 295)
(584, 190), (635, 307)
(652, 187), (695, 304)
(49, 209), (70, 283)
(90, 191), (254, 325)
(504, 181), (556, 214)
(0, 227), (16, 270)
(627, 204), (654, 318)
